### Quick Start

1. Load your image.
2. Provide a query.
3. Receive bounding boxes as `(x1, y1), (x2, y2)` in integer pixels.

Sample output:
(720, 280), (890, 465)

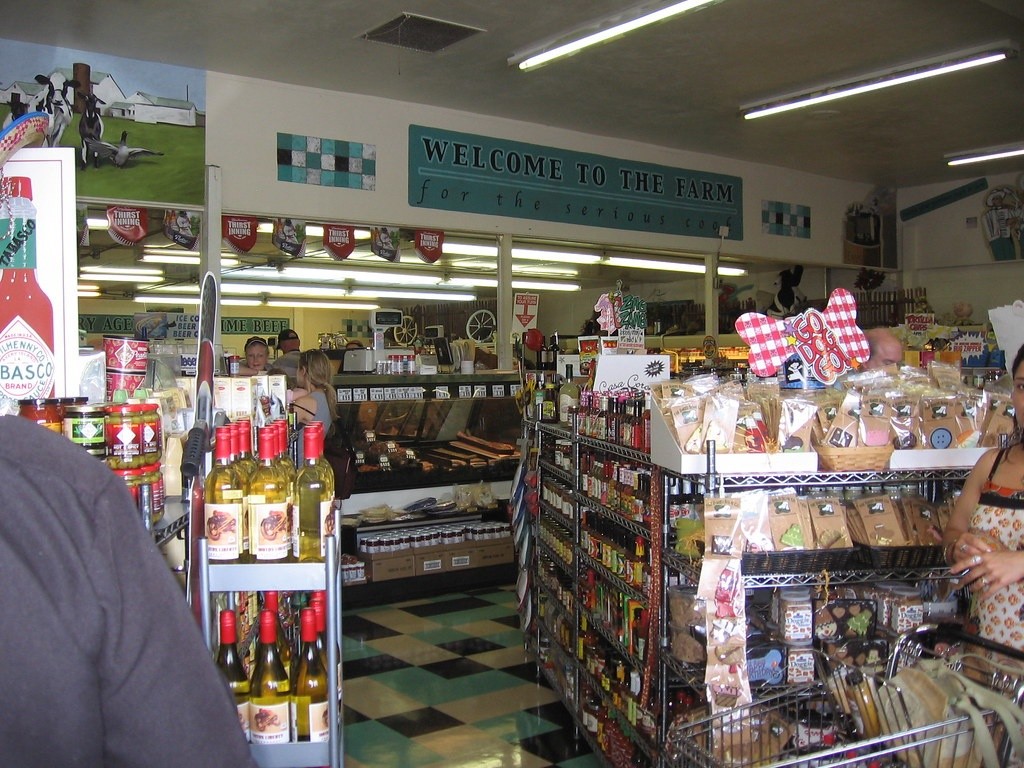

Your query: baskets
(853, 540), (944, 570)
(746, 546), (861, 573)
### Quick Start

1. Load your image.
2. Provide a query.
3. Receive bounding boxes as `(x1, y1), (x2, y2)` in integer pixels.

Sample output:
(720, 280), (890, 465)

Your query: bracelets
(942, 541), (956, 568)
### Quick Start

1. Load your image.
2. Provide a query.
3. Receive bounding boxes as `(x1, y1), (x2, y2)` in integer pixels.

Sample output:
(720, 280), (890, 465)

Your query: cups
(376, 360), (385, 374)
(461, 361), (474, 375)
(385, 360), (395, 374)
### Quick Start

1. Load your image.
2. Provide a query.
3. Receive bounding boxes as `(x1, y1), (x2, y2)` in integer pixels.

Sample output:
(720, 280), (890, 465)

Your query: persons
(290, 350), (340, 471)
(860, 326), (903, 376)
(0, 416), (258, 768)
(941, 344), (1024, 694)
(267, 369), (308, 405)
(237, 337), (276, 375)
(270, 328), (307, 378)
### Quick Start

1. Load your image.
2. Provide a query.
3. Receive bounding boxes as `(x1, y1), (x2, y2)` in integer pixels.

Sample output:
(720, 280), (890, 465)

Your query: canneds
(341, 553), (367, 580)
(359, 521), (511, 553)
(17, 396), (165, 524)
(566, 405), (579, 428)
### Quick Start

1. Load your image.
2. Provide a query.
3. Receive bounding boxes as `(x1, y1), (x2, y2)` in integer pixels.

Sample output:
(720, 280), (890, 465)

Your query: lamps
(945, 137), (1024, 171)
(129, 293), (264, 307)
(78, 284), (101, 291)
(508, 0), (727, 78)
(737, 40), (1018, 123)
(79, 265), (166, 275)
(134, 282), (347, 301)
(346, 288), (479, 301)
(439, 236), (602, 266)
(256, 221), (371, 243)
(440, 273), (584, 294)
(78, 290), (102, 297)
(84, 210), (111, 231)
(77, 273), (168, 283)
(223, 264), (445, 291)
(134, 248), (244, 268)
(264, 297), (382, 310)
(602, 248), (750, 279)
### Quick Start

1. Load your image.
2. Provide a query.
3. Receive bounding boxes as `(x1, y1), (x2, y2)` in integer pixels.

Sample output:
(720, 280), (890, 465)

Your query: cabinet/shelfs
(155, 340), (974, 768)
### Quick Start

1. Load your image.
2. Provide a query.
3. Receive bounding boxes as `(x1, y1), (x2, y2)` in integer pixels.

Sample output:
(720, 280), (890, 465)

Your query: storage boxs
(213, 378), (233, 421)
(477, 533), (515, 564)
(233, 376), (258, 421)
(889, 446), (996, 467)
(444, 539), (477, 570)
(359, 548), (416, 582)
(252, 374), (269, 402)
(415, 541), (447, 576)
(269, 374), (288, 408)
(648, 395), (819, 473)
(177, 377), (190, 392)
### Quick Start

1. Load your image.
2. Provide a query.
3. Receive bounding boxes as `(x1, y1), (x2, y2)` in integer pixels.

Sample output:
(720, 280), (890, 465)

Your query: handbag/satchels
(879, 654), (1024, 768)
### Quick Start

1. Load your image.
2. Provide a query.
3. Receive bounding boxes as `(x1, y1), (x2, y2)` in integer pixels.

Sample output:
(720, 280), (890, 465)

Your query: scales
(423, 325), (444, 343)
(342, 309), (415, 373)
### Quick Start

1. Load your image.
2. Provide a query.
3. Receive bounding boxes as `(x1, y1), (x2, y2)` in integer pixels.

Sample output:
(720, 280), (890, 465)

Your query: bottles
(0, 177), (57, 417)
(203, 416), (345, 744)
(844, 672), (878, 738)
(17, 394), (165, 529)
(342, 522), (510, 582)
(973, 368), (1007, 389)
(389, 354), (416, 375)
(529, 331), (750, 767)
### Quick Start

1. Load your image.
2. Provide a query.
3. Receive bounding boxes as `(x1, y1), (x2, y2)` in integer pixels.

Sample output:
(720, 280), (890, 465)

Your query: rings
(960, 544), (967, 550)
(981, 576), (987, 584)
(974, 557), (979, 565)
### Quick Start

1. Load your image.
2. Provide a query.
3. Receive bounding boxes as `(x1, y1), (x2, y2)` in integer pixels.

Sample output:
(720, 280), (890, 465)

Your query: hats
(275, 328), (299, 350)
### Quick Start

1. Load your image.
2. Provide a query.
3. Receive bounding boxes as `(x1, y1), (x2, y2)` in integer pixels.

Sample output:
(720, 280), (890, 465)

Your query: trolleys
(660, 619), (1024, 768)
